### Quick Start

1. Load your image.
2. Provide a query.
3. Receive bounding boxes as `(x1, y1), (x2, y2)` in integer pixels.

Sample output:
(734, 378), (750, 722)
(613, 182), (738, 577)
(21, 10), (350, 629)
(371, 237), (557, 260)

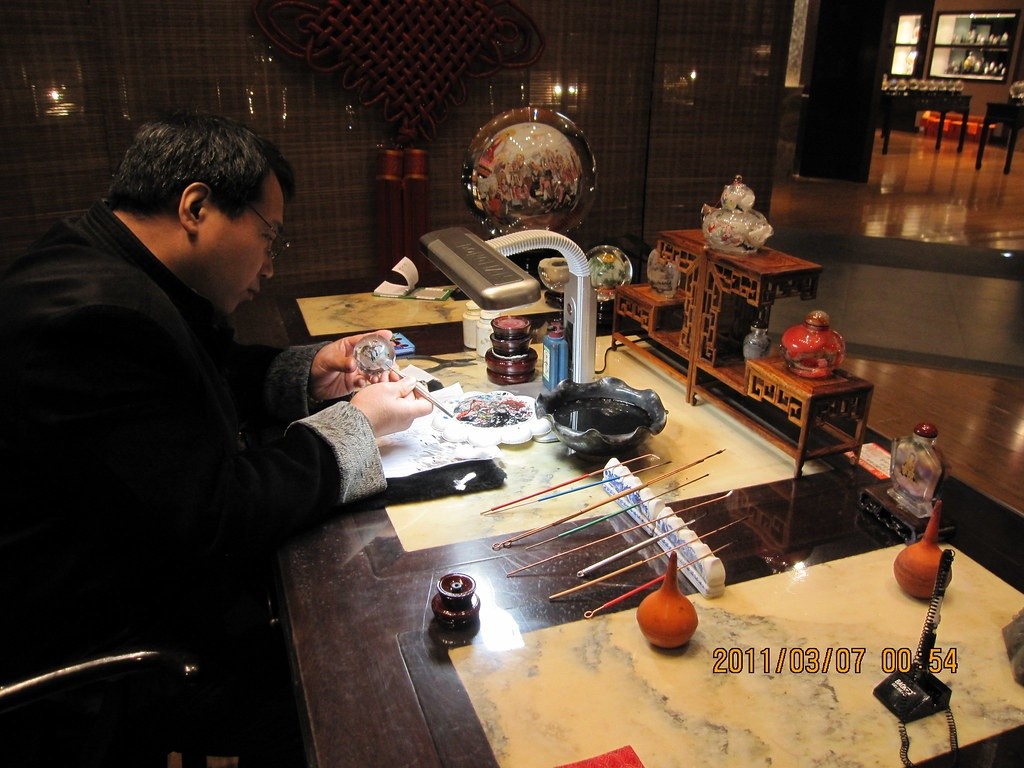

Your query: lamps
(418, 226), (595, 384)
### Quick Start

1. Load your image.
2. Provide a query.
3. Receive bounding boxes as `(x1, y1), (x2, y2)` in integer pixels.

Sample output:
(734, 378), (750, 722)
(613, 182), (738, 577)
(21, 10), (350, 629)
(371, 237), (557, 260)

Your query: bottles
(477, 310), (501, 359)
(462, 301), (484, 350)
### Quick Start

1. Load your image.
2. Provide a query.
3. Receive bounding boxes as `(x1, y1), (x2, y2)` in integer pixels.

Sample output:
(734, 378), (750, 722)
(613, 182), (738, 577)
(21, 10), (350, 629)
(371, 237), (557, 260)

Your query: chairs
(0, 640), (200, 767)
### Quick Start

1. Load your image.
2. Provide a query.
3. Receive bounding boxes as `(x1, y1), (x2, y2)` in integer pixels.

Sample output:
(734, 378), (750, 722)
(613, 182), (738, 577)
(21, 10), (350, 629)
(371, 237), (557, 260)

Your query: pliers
(406, 354), (478, 374)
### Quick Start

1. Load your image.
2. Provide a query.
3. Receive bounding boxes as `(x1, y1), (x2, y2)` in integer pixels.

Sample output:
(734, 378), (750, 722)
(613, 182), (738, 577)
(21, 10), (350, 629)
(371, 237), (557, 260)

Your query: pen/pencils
(913, 548), (954, 682)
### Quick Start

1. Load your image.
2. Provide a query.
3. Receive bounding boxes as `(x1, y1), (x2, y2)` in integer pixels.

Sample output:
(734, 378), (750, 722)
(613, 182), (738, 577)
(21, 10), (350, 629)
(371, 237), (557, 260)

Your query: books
(371, 255), (454, 302)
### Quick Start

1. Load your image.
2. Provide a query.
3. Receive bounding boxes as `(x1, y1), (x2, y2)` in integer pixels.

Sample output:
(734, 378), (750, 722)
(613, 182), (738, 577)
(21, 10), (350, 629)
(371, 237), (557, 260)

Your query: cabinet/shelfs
(611, 228), (875, 480)
(929, 43), (1011, 84)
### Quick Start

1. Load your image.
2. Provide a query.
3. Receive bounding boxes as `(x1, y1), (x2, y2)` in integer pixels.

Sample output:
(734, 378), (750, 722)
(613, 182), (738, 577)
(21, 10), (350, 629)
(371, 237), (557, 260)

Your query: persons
(0, 109), (433, 768)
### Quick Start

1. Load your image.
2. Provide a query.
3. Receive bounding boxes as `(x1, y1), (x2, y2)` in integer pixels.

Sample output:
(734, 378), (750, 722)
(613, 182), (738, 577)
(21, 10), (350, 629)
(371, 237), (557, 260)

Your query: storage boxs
(921, 110), (996, 146)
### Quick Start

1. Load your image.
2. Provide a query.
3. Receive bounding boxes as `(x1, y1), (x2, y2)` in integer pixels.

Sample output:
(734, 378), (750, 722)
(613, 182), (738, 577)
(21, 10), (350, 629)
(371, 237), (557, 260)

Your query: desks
(975, 101), (1024, 175)
(879, 96), (973, 152)
(275, 271), (1024, 768)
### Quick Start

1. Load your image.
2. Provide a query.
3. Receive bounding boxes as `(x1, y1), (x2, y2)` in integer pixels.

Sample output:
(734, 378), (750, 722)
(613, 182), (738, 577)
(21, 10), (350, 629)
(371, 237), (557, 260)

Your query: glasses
(251, 206), (291, 260)
